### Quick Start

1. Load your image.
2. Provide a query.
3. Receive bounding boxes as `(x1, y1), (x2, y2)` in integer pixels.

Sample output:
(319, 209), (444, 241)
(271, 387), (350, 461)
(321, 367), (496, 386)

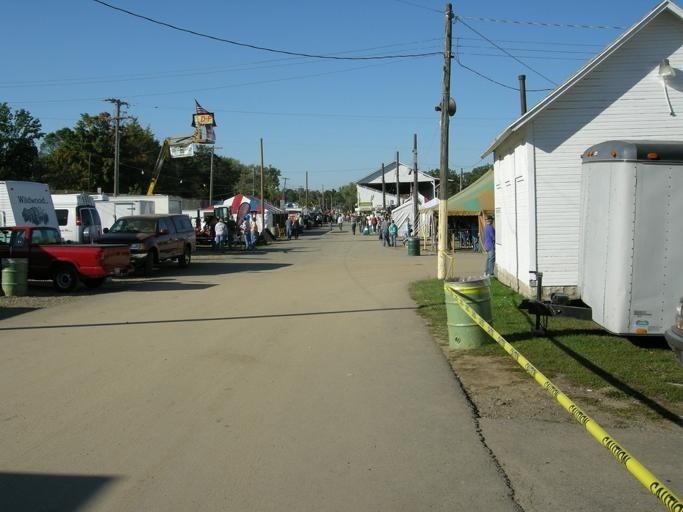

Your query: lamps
(656, 56), (677, 117)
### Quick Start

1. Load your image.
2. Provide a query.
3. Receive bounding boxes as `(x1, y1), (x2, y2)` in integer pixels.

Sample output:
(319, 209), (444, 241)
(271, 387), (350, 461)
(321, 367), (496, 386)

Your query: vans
(51, 193), (101, 242)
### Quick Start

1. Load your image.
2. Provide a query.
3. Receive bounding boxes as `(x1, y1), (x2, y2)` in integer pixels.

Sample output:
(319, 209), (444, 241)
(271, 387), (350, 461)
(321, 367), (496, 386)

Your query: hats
(484, 215), (493, 220)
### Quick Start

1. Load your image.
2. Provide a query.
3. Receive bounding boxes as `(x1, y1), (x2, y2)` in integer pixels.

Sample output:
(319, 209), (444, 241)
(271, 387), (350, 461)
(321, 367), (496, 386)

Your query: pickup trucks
(0, 226), (133, 292)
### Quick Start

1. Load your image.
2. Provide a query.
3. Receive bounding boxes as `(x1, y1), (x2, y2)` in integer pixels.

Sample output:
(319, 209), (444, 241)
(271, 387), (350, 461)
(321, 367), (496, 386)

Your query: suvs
(99, 214), (196, 272)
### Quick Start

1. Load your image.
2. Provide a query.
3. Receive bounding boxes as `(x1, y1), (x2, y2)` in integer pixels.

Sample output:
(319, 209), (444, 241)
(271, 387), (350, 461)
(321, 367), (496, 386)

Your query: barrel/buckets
(271, 227), (276, 236)
(408, 237), (420, 257)
(444, 277), (493, 351)
(2, 257), (28, 297)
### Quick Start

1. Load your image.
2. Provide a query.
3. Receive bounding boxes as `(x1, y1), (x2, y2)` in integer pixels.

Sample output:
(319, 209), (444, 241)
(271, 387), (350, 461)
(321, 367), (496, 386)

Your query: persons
(193, 214), (257, 252)
(402, 217), (414, 245)
(283, 212), (398, 247)
(483, 214), (494, 275)
